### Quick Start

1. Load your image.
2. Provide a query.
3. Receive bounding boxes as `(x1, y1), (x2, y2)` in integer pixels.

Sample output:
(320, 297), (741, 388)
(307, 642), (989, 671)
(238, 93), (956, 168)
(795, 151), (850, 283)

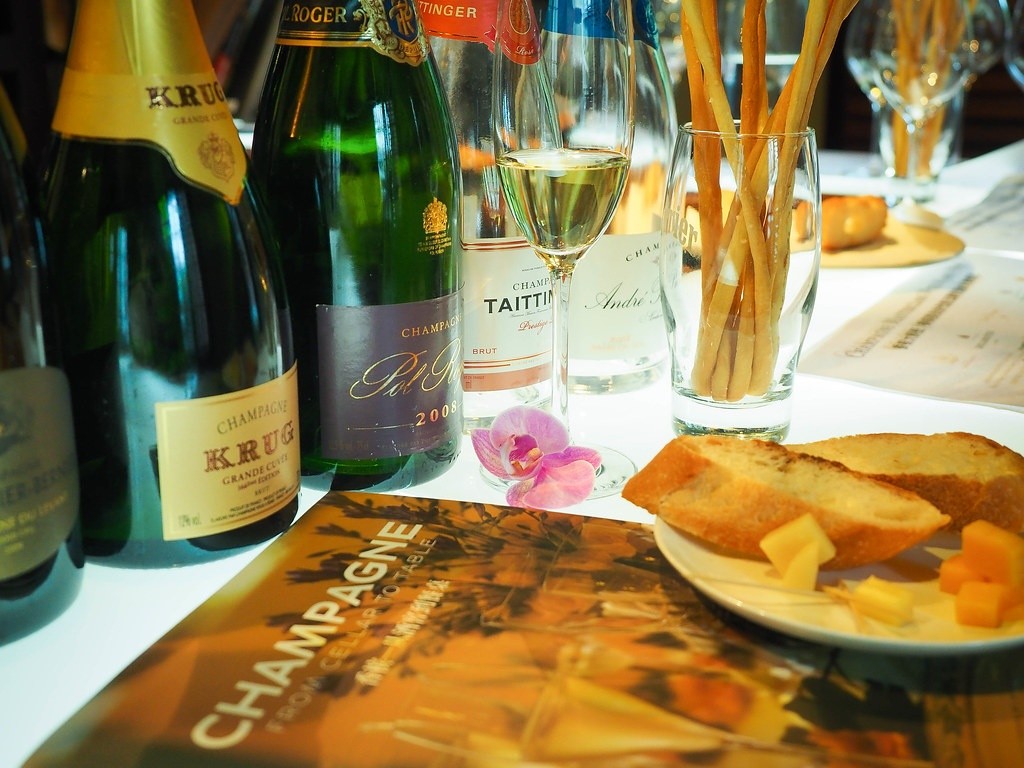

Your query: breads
(781, 432), (1024, 539)
(689, 193), (888, 250)
(622, 438), (950, 567)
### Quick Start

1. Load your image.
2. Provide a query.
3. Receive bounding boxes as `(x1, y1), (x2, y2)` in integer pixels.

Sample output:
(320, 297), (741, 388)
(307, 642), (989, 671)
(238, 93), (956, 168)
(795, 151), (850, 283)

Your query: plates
(654, 516), (1024, 654)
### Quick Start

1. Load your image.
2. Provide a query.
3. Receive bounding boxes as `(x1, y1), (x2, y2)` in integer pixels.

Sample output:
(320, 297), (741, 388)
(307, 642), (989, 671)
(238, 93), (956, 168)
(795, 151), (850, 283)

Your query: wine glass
(496, 0), (637, 503)
(848, 0), (1024, 228)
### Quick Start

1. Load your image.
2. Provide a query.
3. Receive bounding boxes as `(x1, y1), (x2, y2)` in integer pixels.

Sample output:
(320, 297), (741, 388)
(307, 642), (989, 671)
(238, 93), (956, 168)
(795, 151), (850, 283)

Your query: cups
(660, 122), (822, 429)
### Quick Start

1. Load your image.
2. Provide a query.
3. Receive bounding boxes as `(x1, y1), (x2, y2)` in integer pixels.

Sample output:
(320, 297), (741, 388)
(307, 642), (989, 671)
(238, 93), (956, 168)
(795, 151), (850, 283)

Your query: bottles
(252, 0), (461, 490)
(33, 0), (299, 566)
(516, 0), (681, 394)
(0, 90), (74, 644)
(413, 0), (560, 436)
